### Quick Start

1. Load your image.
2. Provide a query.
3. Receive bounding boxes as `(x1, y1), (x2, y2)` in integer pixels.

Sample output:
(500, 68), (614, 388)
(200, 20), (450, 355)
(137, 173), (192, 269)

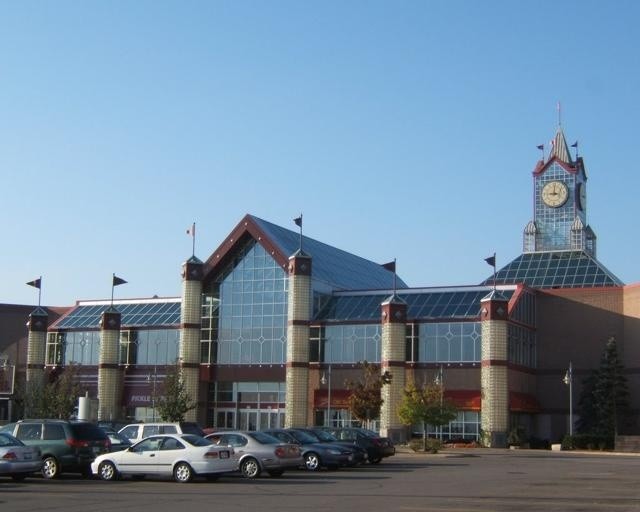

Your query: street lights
(321, 365), (331, 425)
(562, 361), (573, 449)
(435, 364), (443, 443)
(146, 369), (156, 419)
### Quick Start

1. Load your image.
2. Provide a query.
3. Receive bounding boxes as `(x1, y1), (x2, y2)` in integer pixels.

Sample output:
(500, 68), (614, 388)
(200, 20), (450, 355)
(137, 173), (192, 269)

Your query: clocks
(579, 182), (586, 211)
(541, 181), (569, 208)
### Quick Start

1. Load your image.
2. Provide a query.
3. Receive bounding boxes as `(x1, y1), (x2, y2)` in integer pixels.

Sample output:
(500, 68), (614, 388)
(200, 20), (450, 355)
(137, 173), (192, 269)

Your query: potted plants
(506, 424), (526, 449)
